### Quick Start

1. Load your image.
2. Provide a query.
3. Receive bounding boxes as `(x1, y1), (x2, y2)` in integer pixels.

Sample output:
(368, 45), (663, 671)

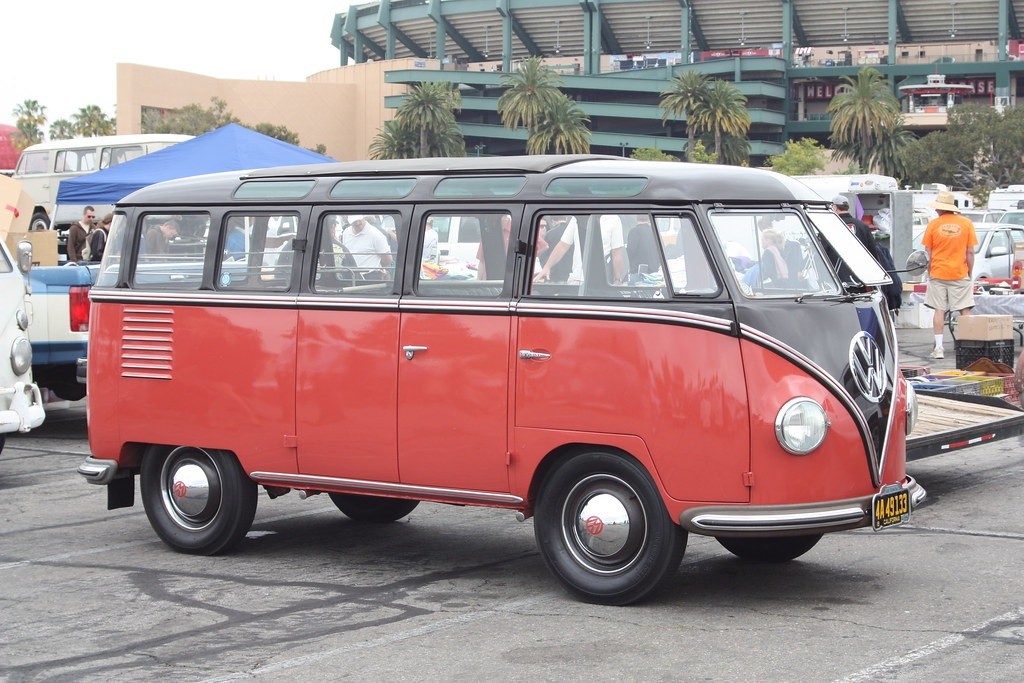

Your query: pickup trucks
(23, 252), (276, 399)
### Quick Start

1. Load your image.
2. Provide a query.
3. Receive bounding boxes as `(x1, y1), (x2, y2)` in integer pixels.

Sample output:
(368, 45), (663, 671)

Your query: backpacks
(81, 228), (106, 260)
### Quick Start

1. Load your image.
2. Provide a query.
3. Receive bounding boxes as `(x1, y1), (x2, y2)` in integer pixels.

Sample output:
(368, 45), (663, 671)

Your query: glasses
(88, 216), (96, 218)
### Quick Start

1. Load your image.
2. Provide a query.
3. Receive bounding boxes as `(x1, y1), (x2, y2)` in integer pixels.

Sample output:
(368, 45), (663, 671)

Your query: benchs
(342, 279), (505, 297)
(613, 287), (669, 297)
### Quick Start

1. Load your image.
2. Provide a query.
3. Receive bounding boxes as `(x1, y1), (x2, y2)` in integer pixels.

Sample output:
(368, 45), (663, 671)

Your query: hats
(927, 190), (961, 214)
(832, 195), (848, 205)
(348, 215), (363, 224)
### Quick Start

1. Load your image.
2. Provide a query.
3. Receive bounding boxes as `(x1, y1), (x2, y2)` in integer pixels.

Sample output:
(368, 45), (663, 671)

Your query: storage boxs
(897, 278), (1021, 406)
(0, 173), (58, 266)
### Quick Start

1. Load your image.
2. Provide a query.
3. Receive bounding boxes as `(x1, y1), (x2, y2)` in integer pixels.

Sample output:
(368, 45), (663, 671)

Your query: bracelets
(614, 278), (622, 281)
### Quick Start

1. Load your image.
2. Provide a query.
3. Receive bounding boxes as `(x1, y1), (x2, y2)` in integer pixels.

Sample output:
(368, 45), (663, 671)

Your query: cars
(914, 211), (1024, 292)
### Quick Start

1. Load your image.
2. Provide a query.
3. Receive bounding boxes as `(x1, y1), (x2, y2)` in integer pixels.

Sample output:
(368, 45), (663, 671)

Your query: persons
(627, 214), (665, 275)
(533, 214), (624, 286)
(90, 213), (113, 261)
(818, 195), (875, 284)
(476, 213), (573, 283)
(145, 214), (439, 288)
(68, 206), (95, 262)
(922, 192), (978, 359)
(761, 230), (784, 289)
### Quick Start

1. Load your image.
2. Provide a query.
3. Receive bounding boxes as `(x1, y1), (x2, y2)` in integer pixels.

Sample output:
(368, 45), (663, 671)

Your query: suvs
(956, 208), (1005, 224)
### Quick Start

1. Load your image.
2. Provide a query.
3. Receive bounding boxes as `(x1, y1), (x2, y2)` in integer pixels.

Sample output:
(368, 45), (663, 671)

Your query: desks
(915, 294), (1024, 349)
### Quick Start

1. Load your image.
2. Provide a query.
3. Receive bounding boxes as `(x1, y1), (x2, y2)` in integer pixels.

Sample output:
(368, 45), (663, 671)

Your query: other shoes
(931, 347), (944, 358)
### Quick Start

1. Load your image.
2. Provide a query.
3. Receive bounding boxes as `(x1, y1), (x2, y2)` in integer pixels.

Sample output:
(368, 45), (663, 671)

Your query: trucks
(793, 174), (913, 278)
(904, 182), (974, 211)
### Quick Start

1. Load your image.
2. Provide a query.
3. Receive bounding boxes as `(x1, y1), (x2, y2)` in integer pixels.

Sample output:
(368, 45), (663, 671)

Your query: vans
(988, 185), (1024, 211)
(77, 153), (927, 606)
(10, 134), (195, 245)
(0, 233), (46, 455)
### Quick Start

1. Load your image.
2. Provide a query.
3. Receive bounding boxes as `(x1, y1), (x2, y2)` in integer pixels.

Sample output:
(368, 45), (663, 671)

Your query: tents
(49, 123), (342, 229)
